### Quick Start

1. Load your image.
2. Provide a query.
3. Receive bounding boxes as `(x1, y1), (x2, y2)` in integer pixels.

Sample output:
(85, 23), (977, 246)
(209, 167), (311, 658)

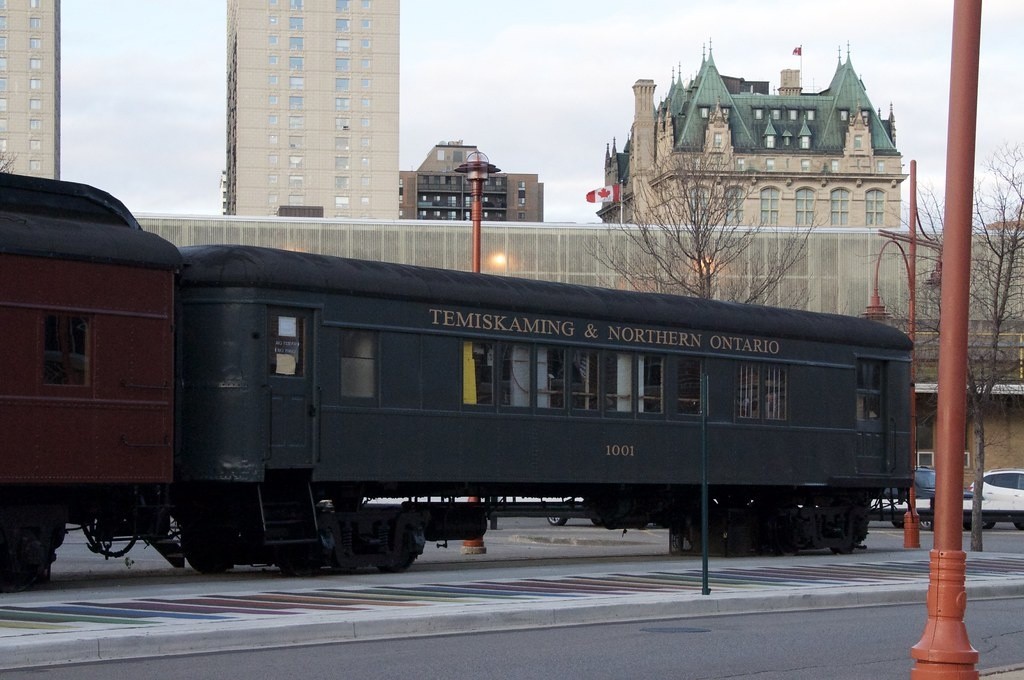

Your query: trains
(1, 173), (914, 590)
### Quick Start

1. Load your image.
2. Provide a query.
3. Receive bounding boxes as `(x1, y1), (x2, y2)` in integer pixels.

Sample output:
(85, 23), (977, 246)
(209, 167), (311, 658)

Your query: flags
(793, 48), (802, 56)
(586, 183), (621, 204)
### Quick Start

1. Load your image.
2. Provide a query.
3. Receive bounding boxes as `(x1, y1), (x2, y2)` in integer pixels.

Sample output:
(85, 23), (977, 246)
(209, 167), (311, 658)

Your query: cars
(963, 468), (1024, 531)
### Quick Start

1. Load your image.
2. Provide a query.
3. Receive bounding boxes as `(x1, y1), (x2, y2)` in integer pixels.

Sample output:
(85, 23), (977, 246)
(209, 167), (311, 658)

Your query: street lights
(869, 467), (987, 531)
(454, 151), (501, 553)
(859, 159), (942, 549)
